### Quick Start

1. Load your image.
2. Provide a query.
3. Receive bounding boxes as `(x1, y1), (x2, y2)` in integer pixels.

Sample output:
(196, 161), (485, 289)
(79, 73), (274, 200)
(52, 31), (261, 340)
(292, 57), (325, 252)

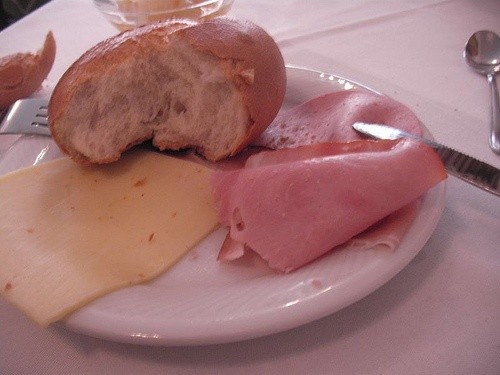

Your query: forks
(0, 98), (52, 136)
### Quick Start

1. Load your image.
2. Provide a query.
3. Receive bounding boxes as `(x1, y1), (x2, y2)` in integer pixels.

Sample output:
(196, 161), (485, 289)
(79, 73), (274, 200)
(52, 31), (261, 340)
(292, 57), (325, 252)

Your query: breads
(46, 15), (287, 166)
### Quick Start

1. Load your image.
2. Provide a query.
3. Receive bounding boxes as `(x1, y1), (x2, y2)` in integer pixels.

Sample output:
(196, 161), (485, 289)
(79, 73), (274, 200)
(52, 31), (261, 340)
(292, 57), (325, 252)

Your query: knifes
(351, 121), (500, 197)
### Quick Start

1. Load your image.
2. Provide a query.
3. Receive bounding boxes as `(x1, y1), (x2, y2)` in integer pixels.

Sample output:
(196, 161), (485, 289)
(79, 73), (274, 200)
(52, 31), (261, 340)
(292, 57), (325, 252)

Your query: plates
(1, 63), (448, 347)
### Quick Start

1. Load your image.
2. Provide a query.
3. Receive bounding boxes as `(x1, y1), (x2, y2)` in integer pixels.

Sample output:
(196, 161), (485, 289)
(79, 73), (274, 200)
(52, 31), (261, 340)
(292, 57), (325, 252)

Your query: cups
(92, 1), (237, 31)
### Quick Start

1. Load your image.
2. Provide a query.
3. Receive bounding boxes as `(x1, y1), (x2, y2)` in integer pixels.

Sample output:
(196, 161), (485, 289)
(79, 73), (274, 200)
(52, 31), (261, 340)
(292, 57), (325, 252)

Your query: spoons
(462, 30), (500, 153)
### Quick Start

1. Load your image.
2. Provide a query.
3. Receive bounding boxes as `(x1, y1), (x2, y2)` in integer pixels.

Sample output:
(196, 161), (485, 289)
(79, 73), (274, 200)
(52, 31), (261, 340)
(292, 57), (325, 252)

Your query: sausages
(216, 90), (447, 271)
